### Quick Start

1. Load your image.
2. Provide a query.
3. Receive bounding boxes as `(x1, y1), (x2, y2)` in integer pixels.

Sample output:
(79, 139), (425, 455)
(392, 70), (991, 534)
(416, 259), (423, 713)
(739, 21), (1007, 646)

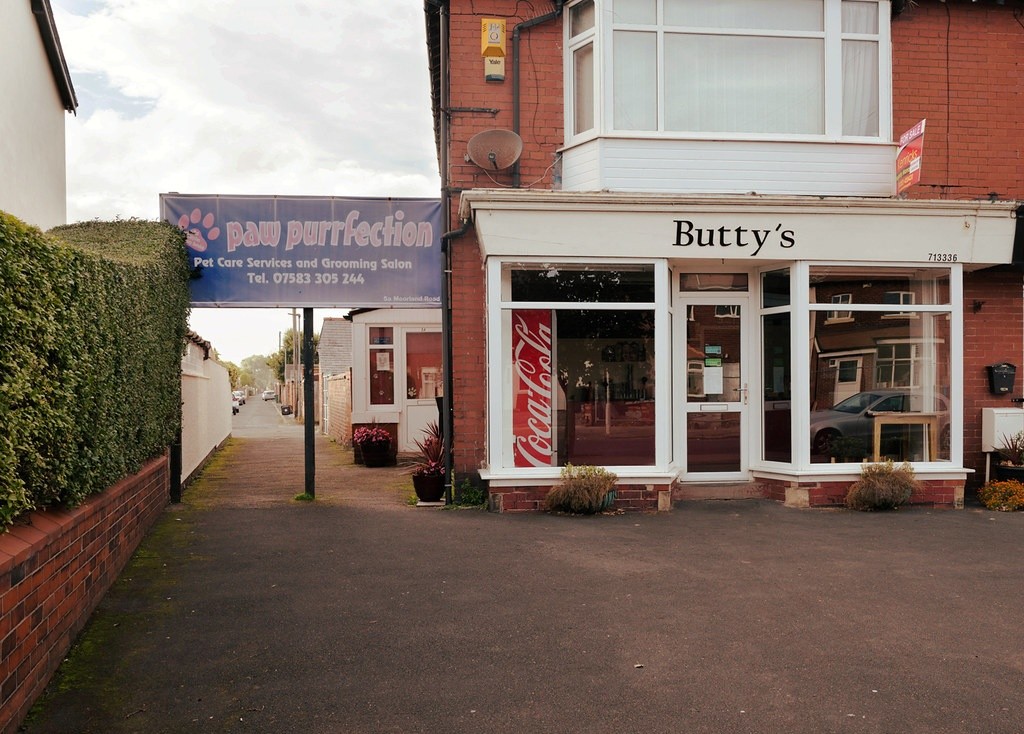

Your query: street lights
(287, 312), (302, 420)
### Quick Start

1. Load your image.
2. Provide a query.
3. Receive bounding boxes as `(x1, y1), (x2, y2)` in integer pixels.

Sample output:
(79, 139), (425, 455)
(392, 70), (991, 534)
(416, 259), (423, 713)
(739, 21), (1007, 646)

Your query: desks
(870, 415), (937, 461)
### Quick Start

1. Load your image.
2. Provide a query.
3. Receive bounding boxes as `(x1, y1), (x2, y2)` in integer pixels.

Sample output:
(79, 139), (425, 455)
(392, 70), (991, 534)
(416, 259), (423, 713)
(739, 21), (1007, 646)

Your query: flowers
(395, 420), (447, 477)
(991, 430), (1024, 467)
(354, 416), (393, 444)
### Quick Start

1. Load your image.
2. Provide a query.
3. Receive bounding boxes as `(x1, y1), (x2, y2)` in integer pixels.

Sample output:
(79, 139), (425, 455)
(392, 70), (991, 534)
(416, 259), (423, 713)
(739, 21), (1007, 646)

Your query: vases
(413, 475), (445, 503)
(995, 465), (1024, 484)
(362, 441), (390, 467)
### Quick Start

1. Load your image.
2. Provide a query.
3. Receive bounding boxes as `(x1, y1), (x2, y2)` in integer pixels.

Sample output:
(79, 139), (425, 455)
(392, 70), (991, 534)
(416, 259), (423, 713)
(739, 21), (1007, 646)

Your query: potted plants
(544, 461), (618, 511)
(847, 455), (934, 514)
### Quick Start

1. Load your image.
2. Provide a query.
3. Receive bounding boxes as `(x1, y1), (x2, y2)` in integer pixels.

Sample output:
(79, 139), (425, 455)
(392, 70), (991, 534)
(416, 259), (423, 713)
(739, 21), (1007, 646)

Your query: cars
(231, 390), (245, 415)
(809, 386), (950, 461)
(261, 390), (275, 401)
